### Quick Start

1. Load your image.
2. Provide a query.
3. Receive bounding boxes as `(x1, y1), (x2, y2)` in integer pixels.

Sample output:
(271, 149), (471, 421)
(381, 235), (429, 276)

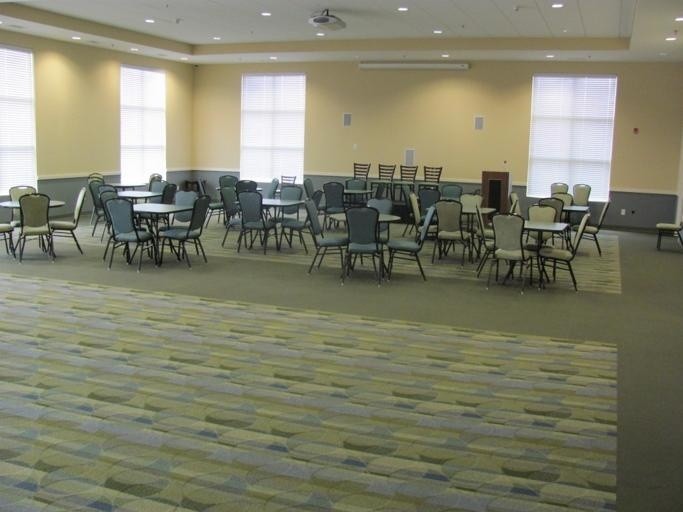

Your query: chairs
(656, 217), (683, 249)
(306, 165), (443, 288)
(218, 172), (310, 253)
(443, 164), (610, 292)
(2, 182), (87, 263)
(88, 171), (210, 269)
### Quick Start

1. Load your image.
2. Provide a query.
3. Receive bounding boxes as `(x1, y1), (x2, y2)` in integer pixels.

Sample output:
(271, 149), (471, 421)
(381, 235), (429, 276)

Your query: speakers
(184, 177), (205, 192)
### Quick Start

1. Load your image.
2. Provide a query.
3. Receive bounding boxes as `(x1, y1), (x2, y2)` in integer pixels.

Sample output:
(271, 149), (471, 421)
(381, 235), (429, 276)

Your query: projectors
(308, 14), (346, 31)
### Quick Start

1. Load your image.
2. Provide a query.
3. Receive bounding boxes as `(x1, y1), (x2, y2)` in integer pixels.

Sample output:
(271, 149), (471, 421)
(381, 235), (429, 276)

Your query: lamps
(308, 8), (337, 26)
(358, 62), (470, 72)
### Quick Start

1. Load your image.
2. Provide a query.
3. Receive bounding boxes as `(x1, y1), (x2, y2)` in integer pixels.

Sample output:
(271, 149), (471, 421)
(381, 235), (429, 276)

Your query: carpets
(0, 270), (621, 512)
(4, 204), (622, 295)
